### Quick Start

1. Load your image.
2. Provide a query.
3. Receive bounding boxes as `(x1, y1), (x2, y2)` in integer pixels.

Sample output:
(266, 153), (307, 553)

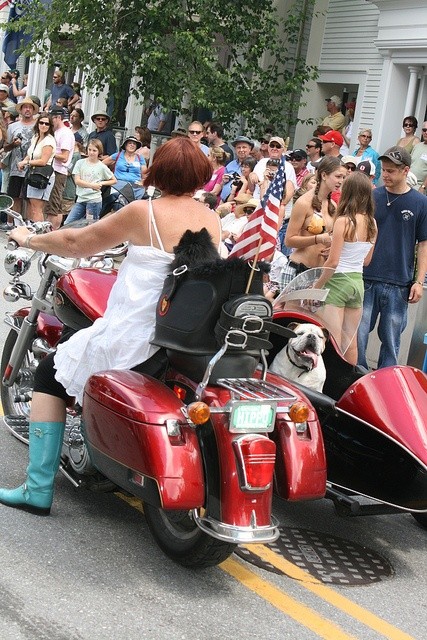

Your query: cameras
(229, 175), (234, 180)
(15, 132), (23, 142)
(268, 171), (276, 177)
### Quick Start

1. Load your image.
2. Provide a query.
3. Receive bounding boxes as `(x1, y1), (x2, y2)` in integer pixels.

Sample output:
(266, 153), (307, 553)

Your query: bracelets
(415, 281), (422, 286)
(314, 235), (316, 245)
(26, 161), (30, 166)
(25, 234), (35, 248)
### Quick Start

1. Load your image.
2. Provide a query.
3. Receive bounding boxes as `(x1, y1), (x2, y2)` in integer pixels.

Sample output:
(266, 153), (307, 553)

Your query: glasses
(360, 135), (371, 138)
(95, 118), (106, 121)
(269, 144), (281, 149)
(345, 165), (355, 171)
(404, 124), (413, 127)
(261, 141), (269, 144)
(422, 129), (427, 132)
(2, 77), (8, 79)
(243, 210), (252, 214)
(306, 144), (316, 149)
(188, 131), (203, 134)
(290, 155), (302, 162)
(38, 121), (50, 126)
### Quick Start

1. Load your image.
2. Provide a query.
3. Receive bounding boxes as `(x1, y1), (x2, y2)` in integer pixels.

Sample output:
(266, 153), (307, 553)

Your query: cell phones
(233, 172), (241, 179)
(302, 299), (321, 306)
(16, 163), (27, 170)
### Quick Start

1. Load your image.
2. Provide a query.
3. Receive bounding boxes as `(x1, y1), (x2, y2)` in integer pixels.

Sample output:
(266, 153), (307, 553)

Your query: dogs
(163, 226), (271, 280)
(269, 321), (330, 394)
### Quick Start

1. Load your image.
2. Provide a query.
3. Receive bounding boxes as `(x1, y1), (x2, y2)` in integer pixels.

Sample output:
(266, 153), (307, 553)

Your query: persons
(226, 156), (257, 212)
(134, 126), (151, 168)
(201, 146), (230, 210)
(69, 108), (88, 155)
(317, 130), (343, 160)
(8, 69), (19, 104)
(60, 132), (82, 224)
(356, 160), (376, 189)
(284, 151), (293, 163)
(396, 116), (421, 154)
(45, 71), (79, 111)
(0, 137), (222, 518)
(16, 113), (57, 223)
(197, 193), (217, 210)
(329, 155), (356, 205)
(11, 74), (28, 103)
(0, 98), (38, 233)
(205, 121), (233, 167)
(187, 121), (209, 157)
(68, 82), (82, 108)
(63, 138), (117, 226)
(219, 136), (254, 205)
(302, 171), (377, 367)
(101, 136), (148, 213)
(338, 101), (356, 157)
(405, 121), (426, 197)
(293, 173), (317, 204)
(259, 134), (271, 158)
(246, 136), (297, 258)
(0, 72), (11, 86)
(0, 84), (15, 122)
(146, 99), (167, 131)
(86, 110), (115, 199)
(28, 95), (40, 119)
(305, 138), (323, 175)
(248, 139), (263, 160)
(288, 149), (309, 188)
(0, 106), (19, 232)
(56, 97), (69, 122)
(312, 94), (345, 136)
(279, 156), (346, 314)
(262, 250), (287, 303)
(351, 129), (380, 185)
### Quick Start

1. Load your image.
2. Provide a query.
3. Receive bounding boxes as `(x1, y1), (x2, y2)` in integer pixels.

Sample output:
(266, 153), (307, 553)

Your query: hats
(8, 69), (19, 77)
(234, 199), (258, 219)
(50, 107), (64, 116)
(120, 135), (142, 150)
(345, 101), (356, 109)
(54, 71), (63, 77)
(377, 146), (411, 169)
(258, 133), (272, 142)
(354, 161), (376, 177)
(318, 130), (344, 148)
(234, 193), (254, 203)
(288, 149), (307, 158)
(268, 136), (285, 148)
(15, 97), (39, 115)
(230, 136), (254, 151)
(2, 106), (19, 116)
(339, 156), (358, 168)
(0, 83), (9, 95)
(91, 109), (111, 125)
(325, 95), (343, 105)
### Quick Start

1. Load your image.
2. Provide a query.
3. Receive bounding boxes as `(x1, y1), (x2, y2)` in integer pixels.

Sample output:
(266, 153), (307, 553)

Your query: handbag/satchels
(24, 164), (54, 189)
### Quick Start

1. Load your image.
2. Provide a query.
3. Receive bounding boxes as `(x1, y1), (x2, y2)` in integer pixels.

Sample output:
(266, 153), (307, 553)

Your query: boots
(0, 421), (66, 517)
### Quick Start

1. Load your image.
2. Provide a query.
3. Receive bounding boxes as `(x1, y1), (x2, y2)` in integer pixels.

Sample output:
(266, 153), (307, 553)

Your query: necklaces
(385, 186), (407, 207)
(125, 153), (134, 160)
(358, 148), (365, 152)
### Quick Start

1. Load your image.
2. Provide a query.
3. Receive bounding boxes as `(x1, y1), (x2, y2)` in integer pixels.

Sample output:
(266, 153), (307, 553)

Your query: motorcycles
(1, 194), (426, 569)
